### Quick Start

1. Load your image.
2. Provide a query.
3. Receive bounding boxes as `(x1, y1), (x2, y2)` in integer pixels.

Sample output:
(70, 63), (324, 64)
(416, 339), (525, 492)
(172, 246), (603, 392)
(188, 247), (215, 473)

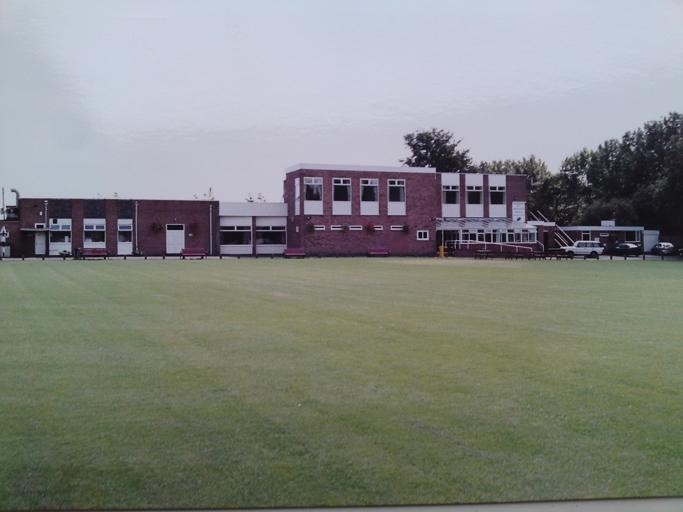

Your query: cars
(609, 242), (641, 257)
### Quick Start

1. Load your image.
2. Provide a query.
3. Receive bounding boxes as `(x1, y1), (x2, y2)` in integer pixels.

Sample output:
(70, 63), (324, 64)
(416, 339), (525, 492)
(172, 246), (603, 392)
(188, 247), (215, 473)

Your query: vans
(559, 240), (604, 258)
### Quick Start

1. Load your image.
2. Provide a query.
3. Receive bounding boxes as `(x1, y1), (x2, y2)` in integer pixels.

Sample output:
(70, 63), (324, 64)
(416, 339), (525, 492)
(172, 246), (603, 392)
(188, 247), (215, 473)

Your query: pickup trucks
(655, 241), (683, 256)
